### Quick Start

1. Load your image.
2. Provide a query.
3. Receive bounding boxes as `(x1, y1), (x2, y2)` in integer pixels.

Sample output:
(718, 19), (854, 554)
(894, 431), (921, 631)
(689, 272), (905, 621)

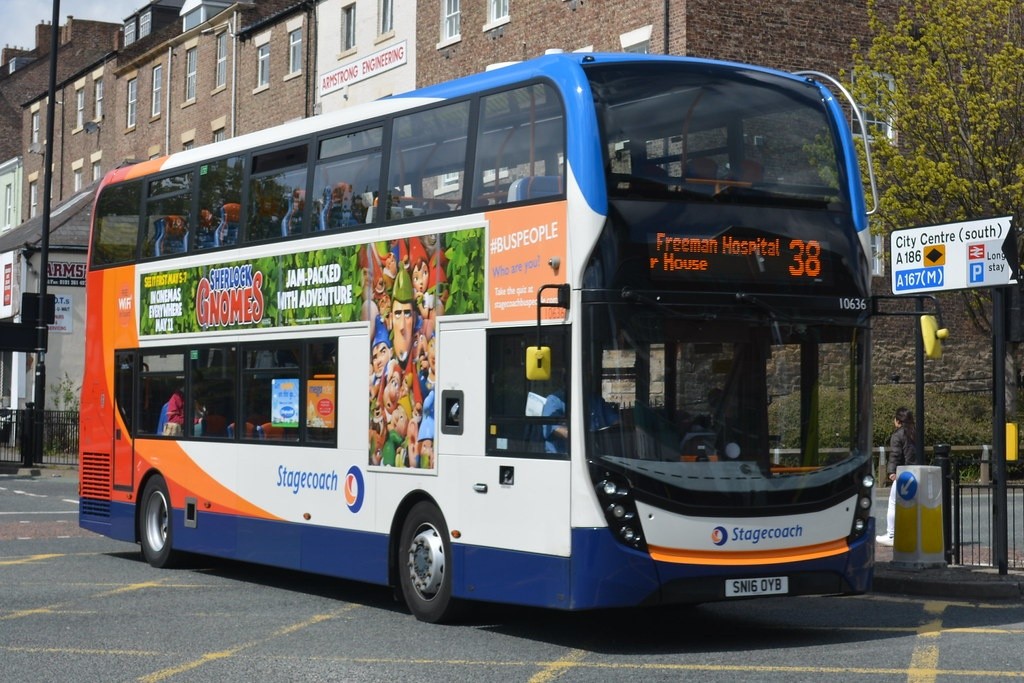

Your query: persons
(542, 388), (620, 454)
(157, 369), (205, 436)
(886, 407), (917, 481)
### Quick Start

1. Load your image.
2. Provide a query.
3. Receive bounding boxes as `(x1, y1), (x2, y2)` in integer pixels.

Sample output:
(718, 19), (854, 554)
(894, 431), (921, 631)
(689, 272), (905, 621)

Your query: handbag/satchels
(163, 422), (181, 436)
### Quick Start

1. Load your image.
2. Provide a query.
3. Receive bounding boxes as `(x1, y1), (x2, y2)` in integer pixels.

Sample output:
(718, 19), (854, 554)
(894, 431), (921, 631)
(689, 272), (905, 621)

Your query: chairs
(227, 423), (254, 438)
(281, 194), (294, 237)
(256, 422), (286, 441)
(318, 186), (333, 230)
(146, 216), (186, 257)
(213, 203), (239, 247)
(508, 176), (563, 203)
(183, 210), (216, 252)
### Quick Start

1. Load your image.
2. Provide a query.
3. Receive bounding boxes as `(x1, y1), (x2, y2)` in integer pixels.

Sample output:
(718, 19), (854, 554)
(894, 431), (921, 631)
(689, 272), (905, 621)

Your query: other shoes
(876, 534), (894, 546)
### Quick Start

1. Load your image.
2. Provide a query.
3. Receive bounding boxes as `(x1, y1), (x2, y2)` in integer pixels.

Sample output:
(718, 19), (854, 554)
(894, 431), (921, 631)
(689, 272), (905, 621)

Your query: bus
(77, 53), (951, 625)
